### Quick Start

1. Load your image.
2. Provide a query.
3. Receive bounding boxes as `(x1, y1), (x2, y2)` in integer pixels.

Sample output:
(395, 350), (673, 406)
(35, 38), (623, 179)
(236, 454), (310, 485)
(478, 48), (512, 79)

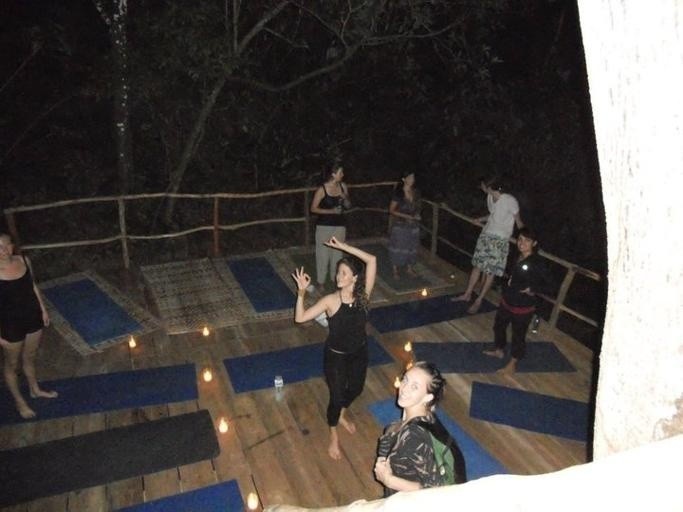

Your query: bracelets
(296, 288), (306, 297)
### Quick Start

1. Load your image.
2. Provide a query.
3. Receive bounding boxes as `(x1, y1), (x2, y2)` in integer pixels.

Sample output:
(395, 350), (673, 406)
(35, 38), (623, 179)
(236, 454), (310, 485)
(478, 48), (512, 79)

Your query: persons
(480, 227), (543, 376)
(0, 231), (59, 422)
(447, 173), (525, 315)
(371, 360), (447, 499)
(385, 164), (424, 280)
(291, 235), (379, 461)
(309, 160), (353, 293)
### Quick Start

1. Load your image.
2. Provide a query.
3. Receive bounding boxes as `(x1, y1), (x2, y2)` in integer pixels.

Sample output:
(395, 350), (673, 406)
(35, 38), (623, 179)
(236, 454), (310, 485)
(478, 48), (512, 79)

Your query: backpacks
(411, 411), (467, 488)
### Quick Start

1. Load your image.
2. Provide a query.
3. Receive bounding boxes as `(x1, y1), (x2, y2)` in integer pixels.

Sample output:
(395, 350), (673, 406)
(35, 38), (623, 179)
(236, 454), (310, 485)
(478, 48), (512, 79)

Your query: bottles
(273, 375), (283, 403)
(530, 316), (540, 335)
(337, 194), (343, 211)
